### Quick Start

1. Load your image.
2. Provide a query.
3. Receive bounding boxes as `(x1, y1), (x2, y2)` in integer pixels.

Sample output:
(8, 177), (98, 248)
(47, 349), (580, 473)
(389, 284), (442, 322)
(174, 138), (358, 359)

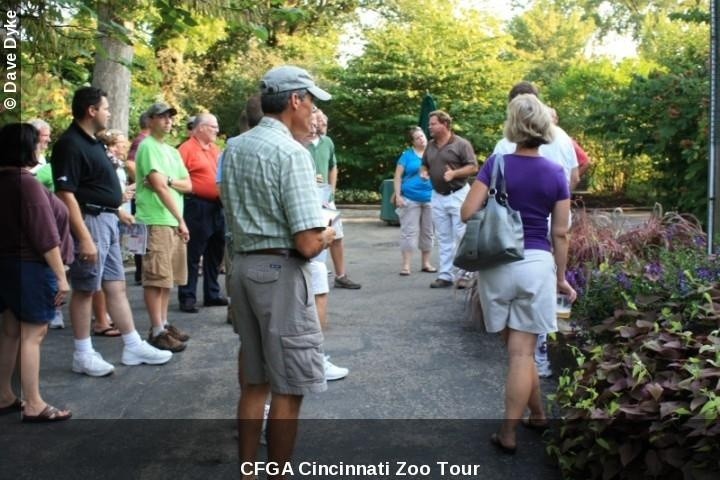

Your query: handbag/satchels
(452, 151), (528, 273)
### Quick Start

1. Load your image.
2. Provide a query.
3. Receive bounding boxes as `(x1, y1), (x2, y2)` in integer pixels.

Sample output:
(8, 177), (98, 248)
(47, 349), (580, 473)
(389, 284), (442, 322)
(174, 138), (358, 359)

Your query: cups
(557, 293), (573, 319)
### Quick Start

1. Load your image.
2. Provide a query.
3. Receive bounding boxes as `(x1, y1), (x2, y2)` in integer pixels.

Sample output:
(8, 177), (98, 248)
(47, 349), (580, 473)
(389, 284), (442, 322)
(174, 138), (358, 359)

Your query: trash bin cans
(379, 179), (400, 226)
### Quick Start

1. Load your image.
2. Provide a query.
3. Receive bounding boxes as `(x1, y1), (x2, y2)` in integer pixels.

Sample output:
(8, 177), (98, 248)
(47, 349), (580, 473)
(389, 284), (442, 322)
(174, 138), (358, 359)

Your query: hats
(257, 64), (333, 103)
(146, 103), (177, 119)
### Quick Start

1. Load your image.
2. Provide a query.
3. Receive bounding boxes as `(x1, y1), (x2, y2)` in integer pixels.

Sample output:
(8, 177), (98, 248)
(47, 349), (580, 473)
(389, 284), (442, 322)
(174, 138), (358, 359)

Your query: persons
(493, 82), (580, 378)
(0, 64), (362, 479)
(394, 125), (438, 276)
(420, 110), (479, 288)
(460, 93), (578, 452)
(548, 106), (592, 177)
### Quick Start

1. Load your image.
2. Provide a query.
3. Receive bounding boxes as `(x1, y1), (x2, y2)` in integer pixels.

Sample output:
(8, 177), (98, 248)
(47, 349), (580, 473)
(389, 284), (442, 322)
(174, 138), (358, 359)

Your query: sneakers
(457, 276), (476, 290)
(179, 303), (202, 315)
(70, 348), (116, 380)
(51, 308), (66, 328)
(236, 399), (273, 447)
(316, 354), (352, 382)
(203, 294), (231, 308)
(429, 278), (455, 290)
(120, 338), (174, 368)
(148, 319), (190, 353)
(335, 273), (363, 290)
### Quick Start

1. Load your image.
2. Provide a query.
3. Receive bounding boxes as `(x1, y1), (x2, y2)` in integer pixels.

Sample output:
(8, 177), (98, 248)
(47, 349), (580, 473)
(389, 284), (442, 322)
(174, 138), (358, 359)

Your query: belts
(80, 202), (118, 217)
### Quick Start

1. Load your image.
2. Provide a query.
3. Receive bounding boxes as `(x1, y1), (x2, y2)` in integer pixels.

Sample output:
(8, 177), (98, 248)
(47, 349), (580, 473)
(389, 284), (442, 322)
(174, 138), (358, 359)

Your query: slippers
(520, 410), (548, 432)
(21, 403), (74, 422)
(96, 323), (123, 339)
(490, 429), (518, 454)
(420, 267), (438, 274)
(399, 264), (413, 278)
(1, 394), (25, 418)
